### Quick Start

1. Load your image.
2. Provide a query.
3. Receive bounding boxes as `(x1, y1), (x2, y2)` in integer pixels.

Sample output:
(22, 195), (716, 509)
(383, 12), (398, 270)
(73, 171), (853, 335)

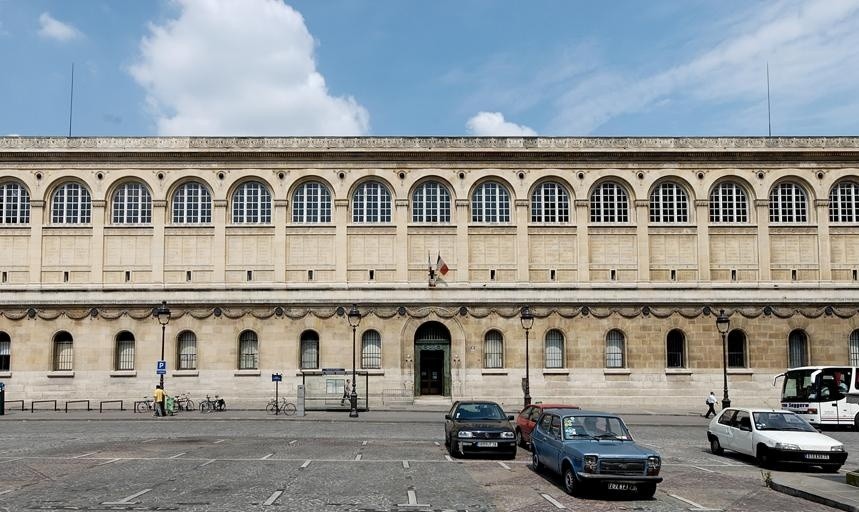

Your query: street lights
(155, 300), (171, 416)
(348, 302), (362, 419)
(715, 308), (732, 410)
(517, 303), (537, 412)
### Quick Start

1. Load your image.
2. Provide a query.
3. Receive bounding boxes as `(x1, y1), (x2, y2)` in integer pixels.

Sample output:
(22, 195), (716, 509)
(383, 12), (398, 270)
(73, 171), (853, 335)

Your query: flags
(436, 256), (450, 277)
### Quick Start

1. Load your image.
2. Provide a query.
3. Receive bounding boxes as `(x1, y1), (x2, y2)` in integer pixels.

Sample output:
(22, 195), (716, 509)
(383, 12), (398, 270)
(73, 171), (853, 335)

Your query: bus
(771, 364), (859, 431)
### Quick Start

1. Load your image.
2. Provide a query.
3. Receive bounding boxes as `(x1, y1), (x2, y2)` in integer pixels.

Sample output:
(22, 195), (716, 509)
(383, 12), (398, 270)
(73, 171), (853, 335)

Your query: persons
(150, 384), (170, 418)
(704, 392), (719, 420)
(339, 378), (352, 407)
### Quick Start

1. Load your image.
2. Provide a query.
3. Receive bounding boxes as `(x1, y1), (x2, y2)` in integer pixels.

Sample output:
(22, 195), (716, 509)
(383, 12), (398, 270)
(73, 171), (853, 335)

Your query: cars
(526, 409), (664, 498)
(444, 399), (518, 459)
(705, 406), (848, 474)
(514, 403), (582, 448)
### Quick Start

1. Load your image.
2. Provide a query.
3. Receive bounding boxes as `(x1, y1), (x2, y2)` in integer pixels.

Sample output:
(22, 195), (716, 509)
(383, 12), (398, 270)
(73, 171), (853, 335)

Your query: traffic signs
(270, 374), (282, 381)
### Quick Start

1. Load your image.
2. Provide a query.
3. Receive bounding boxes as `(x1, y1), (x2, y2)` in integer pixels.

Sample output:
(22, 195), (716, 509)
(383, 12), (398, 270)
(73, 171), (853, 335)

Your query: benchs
(381, 388), (415, 407)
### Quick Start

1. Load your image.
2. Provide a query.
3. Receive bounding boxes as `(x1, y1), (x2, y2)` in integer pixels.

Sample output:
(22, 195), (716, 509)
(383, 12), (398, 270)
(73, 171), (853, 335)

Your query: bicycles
(135, 394), (228, 415)
(265, 396), (297, 416)
